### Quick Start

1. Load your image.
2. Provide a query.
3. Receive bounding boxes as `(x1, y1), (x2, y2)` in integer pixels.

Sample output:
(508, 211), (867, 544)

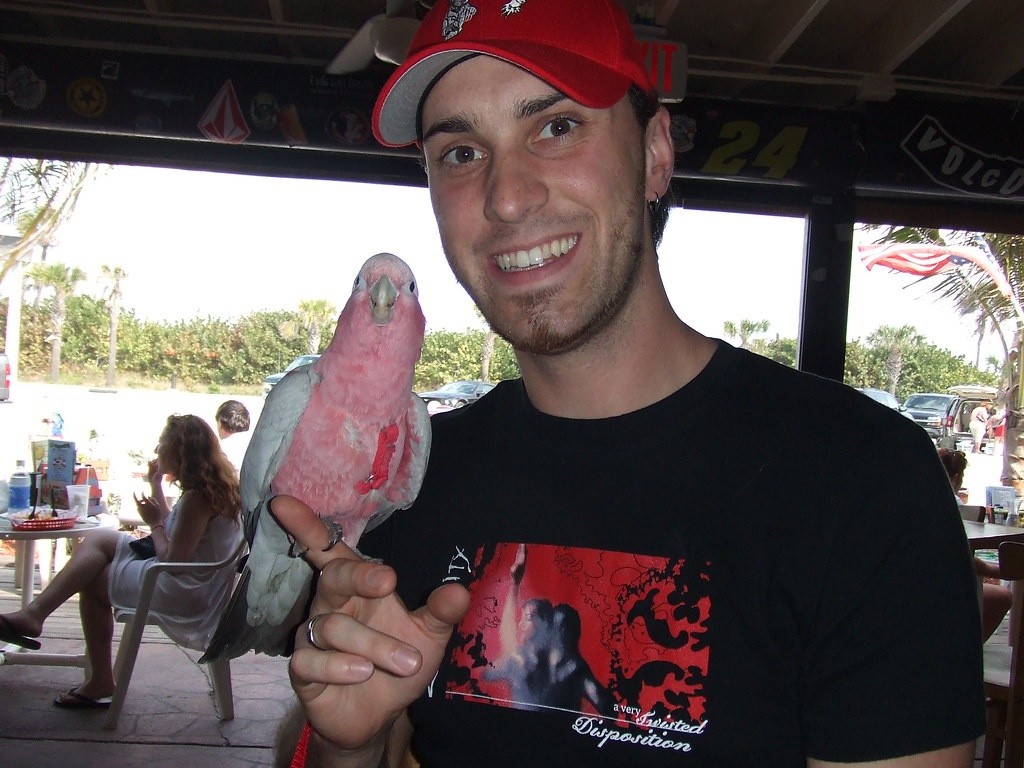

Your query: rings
(305, 614), (326, 651)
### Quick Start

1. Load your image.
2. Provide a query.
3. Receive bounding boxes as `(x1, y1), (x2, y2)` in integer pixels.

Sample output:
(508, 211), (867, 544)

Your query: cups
(66, 485), (90, 523)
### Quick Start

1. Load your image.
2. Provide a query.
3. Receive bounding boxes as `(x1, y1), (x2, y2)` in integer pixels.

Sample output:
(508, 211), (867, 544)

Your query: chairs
(982, 541), (1024, 767)
(102, 537), (250, 730)
(960, 505), (986, 522)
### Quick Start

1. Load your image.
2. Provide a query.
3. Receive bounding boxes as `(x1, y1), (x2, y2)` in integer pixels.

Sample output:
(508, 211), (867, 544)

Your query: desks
(962, 519), (1024, 551)
(0, 511), (121, 683)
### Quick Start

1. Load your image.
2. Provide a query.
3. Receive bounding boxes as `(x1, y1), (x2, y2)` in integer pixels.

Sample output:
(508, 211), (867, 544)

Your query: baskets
(9, 508), (75, 531)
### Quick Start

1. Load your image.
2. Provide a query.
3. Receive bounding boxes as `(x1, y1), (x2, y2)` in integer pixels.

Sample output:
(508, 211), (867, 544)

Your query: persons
(215, 400), (252, 484)
(970, 402), (1006, 455)
(934, 448), (1011, 641)
(273, 0), (983, 766)
(1, 416), (249, 708)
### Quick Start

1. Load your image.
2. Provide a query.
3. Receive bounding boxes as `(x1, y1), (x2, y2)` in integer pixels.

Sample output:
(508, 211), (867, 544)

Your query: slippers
(54, 686), (112, 708)
(0, 615), (42, 650)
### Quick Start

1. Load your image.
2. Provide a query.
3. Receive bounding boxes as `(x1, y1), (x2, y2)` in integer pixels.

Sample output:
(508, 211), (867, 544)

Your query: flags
(857, 232), (1015, 301)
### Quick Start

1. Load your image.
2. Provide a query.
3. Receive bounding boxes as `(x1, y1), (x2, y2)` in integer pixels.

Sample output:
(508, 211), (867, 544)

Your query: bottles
(8, 460), (30, 515)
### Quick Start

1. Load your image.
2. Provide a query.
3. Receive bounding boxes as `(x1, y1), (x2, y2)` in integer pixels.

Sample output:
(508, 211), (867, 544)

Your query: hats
(371, 0), (686, 150)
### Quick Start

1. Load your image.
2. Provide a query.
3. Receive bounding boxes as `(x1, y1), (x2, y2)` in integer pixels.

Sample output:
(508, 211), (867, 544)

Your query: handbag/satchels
(129, 534), (156, 558)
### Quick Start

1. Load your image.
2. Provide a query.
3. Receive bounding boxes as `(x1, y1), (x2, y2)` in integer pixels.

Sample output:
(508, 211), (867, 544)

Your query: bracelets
(150, 525), (165, 531)
